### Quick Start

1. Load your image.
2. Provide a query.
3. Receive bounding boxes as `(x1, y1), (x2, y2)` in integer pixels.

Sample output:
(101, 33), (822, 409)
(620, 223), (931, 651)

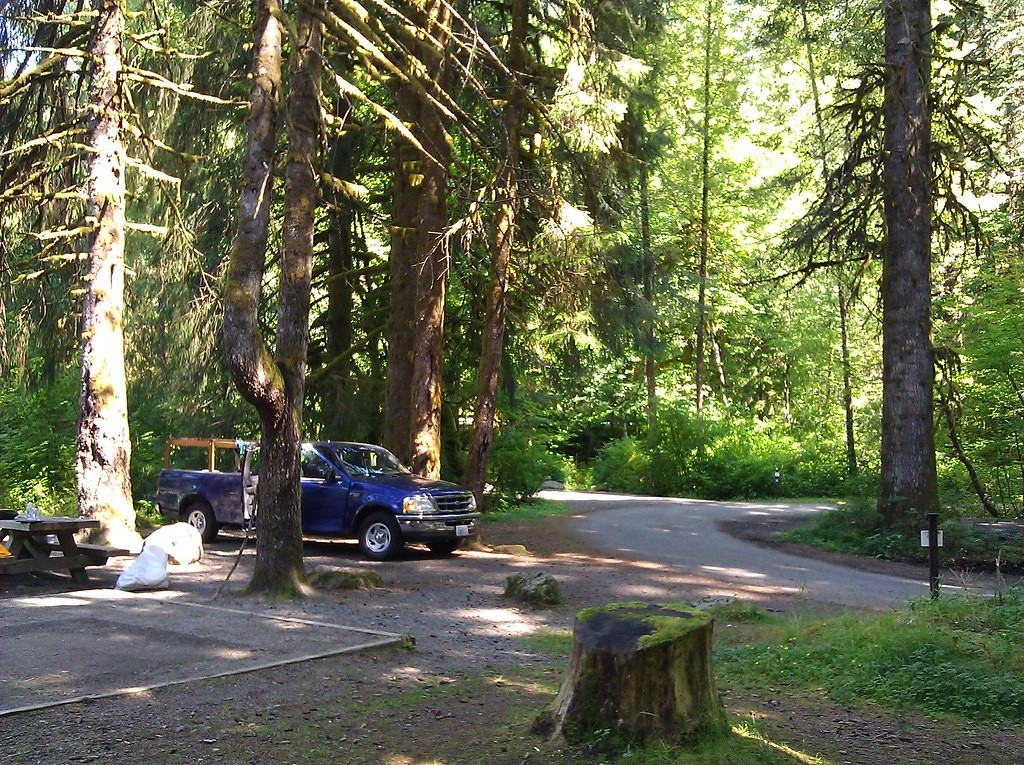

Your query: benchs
(38, 541), (131, 567)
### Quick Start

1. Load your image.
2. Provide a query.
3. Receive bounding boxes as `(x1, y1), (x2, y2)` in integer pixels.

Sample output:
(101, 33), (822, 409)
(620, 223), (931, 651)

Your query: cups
(35, 510), (41, 521)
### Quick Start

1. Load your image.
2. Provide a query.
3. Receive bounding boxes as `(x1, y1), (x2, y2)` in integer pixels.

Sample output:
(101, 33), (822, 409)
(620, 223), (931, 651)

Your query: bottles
(26, 501), (33, 520)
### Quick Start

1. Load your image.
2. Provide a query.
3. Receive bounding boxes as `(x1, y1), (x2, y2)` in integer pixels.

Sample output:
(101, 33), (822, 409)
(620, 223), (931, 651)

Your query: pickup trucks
(157, 437), (481, 561)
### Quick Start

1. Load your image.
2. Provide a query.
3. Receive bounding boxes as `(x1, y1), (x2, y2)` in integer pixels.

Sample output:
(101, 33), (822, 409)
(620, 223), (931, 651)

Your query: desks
(0, 514), (101, 585)
